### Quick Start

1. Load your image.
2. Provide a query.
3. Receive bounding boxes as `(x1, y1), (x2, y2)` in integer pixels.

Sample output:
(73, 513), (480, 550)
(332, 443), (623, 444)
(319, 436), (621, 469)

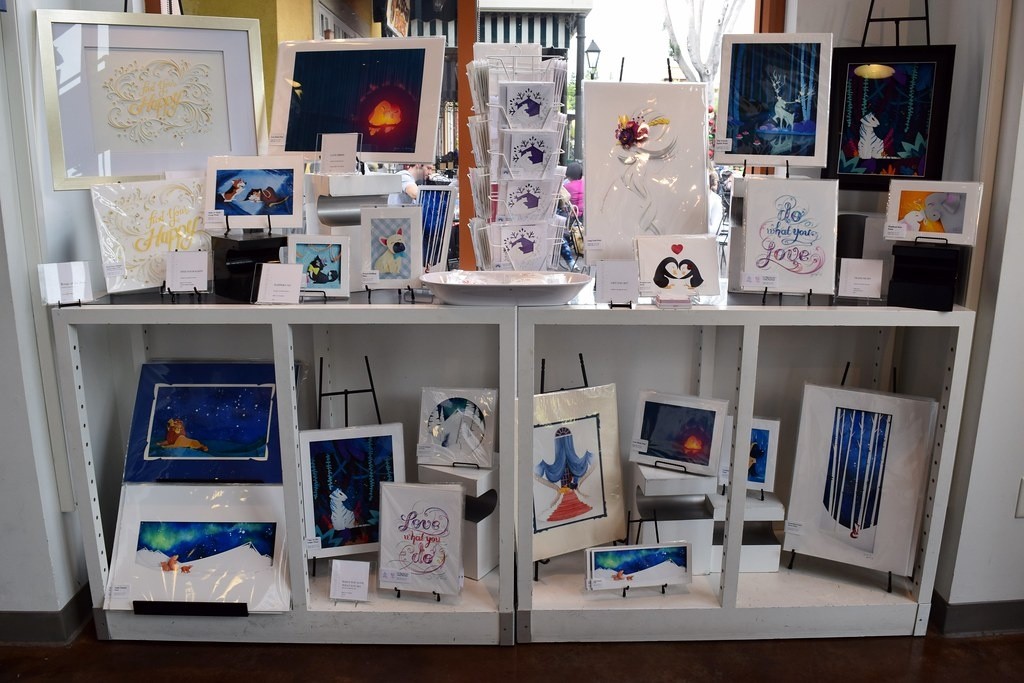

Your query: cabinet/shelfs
(49, 278), (979, 647)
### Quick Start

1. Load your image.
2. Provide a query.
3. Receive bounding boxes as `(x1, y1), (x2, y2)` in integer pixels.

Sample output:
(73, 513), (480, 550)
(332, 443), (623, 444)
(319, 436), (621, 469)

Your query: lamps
(585, 40), (601, 78)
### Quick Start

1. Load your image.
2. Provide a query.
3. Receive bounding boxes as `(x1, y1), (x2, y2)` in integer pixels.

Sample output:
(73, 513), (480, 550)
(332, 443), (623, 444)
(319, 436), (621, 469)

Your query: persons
(563, 165), (585, 225)
(708, 160), (733, 235)
(387, 162), (432, 206)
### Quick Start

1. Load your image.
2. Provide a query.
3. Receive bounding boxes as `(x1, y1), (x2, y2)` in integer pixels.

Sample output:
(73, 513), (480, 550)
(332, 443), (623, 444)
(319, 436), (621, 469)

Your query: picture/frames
(747, 418), (780, 492)
(629, 390), (729, 476)
(207, 155), (302, 229)
(269, 35), (447, 164)
(34, 9), (269, 191)
(360, 206), (421, 290)
(416, 184), (459, 272)
(883, 179), (984, 248)
(714, 31), (834, 168)
(288, 234), (351, 298)
(299, 422), (406, 559)
